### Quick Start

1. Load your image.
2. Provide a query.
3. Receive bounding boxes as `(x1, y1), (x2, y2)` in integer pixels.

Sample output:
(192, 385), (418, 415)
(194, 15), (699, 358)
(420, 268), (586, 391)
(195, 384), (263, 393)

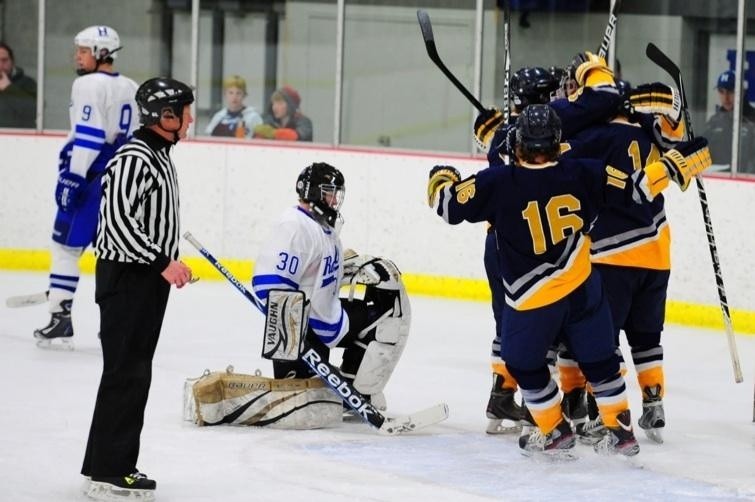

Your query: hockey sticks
(646, 42), (743, 385)
(183, 231), (448, 437)
(416, 9), (485, 115)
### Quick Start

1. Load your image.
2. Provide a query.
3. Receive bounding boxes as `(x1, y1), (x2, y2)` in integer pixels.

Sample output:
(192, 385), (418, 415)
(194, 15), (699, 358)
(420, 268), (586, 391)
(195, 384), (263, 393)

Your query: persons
(485, 66), (564, 434)
(185, 162), (411, 429)
(79, 77), (195, 502)
(472, 52), (684, 460)
(428, 104), (712, 471)
(0, 42), (37, 129)
(206, 76), (263, 139)
(253, 86), (313, 142)
(703, 71), (755, 174)
(34, 25), (142, 348)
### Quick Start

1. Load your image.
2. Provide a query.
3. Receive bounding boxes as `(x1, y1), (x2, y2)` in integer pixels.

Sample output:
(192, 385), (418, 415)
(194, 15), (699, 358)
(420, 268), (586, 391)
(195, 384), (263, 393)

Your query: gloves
(471, 109), (506, 153)
(336, 247), (402, 286)
(255, 122), (277, 140)
(426, 163), (461, 207)
(259, 284), (311, 365)
(624, 80), (683, 127)
(663, 135), (715, 191)
(570, 50), (609, 86)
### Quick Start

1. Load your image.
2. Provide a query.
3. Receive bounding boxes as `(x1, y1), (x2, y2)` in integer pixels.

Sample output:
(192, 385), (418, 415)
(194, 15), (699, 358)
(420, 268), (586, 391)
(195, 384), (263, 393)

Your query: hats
(711, 71), (736, 91)
(277, 85), (302, 119)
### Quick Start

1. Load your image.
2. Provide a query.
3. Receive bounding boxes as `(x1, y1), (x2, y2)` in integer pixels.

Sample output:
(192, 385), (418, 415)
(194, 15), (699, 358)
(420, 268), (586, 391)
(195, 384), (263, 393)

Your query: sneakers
(92, 469), (158, 492)
(482, 373), (534, 421)
(517, 419), (575, 452)
(592, 408), (640, 457)
(33, 298), (75, 340)
(572, 416), (610, 437)
(638, 383), (667, 429)
(561, 386), (589, 421)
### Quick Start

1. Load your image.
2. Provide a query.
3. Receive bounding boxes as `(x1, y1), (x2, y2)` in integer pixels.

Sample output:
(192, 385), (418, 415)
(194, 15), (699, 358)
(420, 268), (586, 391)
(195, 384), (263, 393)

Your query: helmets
(72, 25), (122, 64)
(515, 103), (563, 154)
(509, 67), (564, 98)
(294, 162), (346, 230)
(135, 75), (194, 126)
